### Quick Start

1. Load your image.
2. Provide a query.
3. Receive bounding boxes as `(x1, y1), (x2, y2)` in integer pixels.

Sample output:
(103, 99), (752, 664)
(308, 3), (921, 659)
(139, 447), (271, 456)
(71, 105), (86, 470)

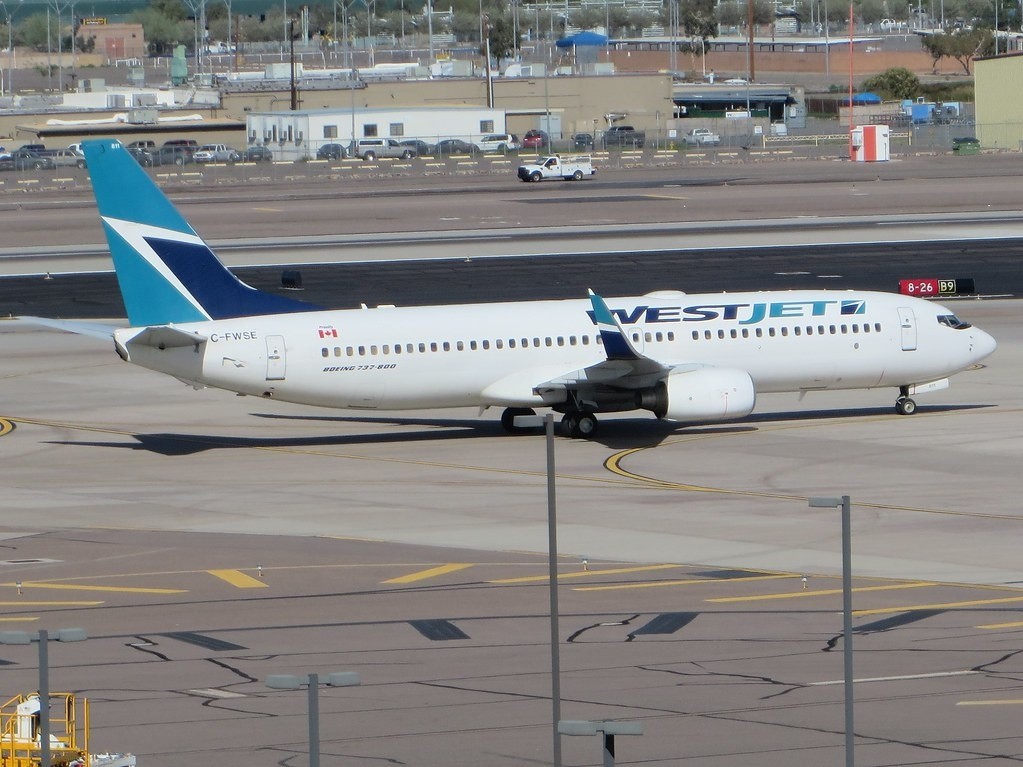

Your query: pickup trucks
(683, 128), (720, 146)
(601, 125), (645, 148)
(518, 153), (597, 182)
(879, 19), (906, 30)
(201, 40), (236, 55)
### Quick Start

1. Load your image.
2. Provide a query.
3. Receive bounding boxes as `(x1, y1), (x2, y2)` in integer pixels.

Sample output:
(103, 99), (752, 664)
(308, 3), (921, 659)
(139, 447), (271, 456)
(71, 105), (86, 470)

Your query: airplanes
(81, 138), (997, 438)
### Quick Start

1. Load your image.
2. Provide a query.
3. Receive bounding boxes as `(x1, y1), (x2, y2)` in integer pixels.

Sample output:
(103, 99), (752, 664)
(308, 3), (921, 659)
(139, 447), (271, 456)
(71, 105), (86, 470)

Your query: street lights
(263, 672), (360, 767)
(557, 720), (643, 767)
(285, 18), (299, 110)
(481, 11), (495, 107)
(809, 495), (855, 767)
(0, 627), (87, 767)
(513, 413), (562, 767)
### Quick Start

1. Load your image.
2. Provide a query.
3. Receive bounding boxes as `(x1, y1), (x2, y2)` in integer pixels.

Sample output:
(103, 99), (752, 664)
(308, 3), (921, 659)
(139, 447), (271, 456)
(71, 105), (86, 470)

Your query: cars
(356, 133), (521, 161)
(572, 133), (595, 150)
(523, 130), (551, 148)
(0, 138), (271, 171)
(317, 144), (346, 160)
